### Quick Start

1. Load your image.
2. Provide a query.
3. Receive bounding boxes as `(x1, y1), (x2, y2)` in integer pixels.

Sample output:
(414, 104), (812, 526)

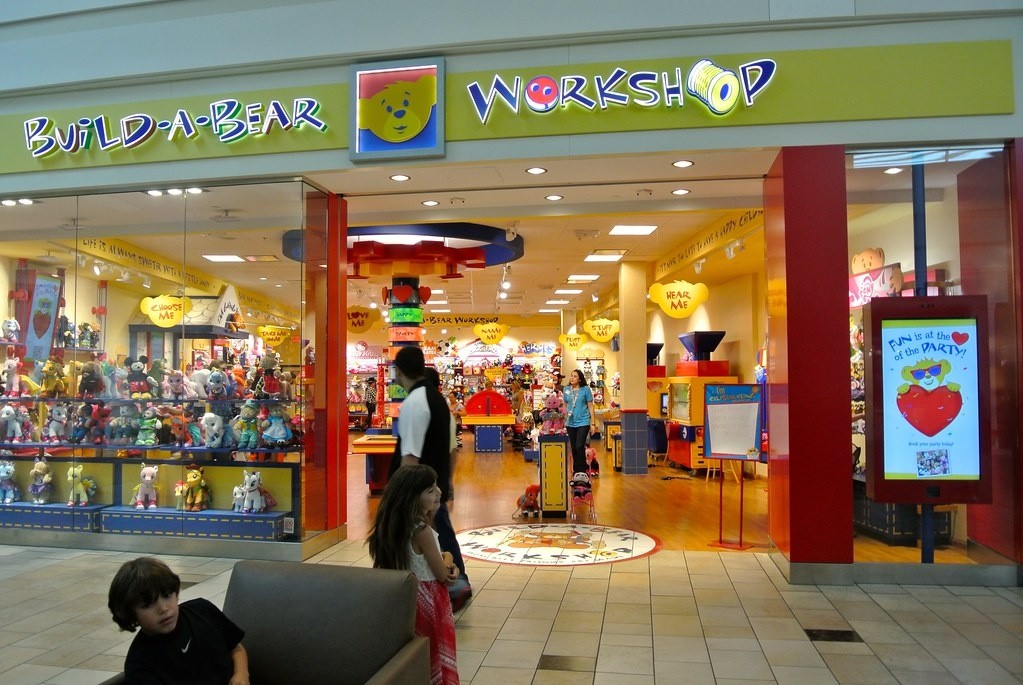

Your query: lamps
(506, 219), (519, 241)
(611, 334), (619, 352)
(78, 253), (152, 289)
(725, 238), (742, 260)
(694, 258), (705, 274)
(291, 323), (296, 330)
(246, 308), (284, 325)
(592, 293), (599, 302)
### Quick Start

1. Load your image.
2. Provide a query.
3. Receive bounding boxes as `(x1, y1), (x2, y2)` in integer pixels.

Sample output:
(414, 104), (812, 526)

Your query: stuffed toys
(348, 357), (623, 517)
(0, 319), (296, 513)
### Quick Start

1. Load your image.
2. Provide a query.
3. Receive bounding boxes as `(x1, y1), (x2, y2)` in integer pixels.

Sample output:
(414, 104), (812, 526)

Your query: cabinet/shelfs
(1, 397), (303, 453)
(493, 367), (531, 397)
(453, 366), (464, 405)
(348, 371), (390, 427)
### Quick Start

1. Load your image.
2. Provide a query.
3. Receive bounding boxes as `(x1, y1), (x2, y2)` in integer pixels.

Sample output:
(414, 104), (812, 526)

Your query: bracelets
(591, 424), (595, 426)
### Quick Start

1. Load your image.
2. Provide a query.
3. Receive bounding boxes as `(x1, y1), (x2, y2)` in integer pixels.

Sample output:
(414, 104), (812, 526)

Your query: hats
(366, 377), (376, 382)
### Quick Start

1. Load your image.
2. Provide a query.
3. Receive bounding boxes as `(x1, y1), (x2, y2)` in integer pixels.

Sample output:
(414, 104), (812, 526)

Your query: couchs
(100, 559), (433, 685)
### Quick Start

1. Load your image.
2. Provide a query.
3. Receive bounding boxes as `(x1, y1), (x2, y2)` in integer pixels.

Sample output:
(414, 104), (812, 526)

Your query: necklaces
(568, 386), (580, 416)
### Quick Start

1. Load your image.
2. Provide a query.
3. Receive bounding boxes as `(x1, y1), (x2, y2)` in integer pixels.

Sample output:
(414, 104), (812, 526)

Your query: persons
(388, 346), (470, 584)
(108, 556), (252, 685)
(562, 368), (597, 477)
(447, 391), (467, 435)
(364, 464), (460, 685)
(360, 377), (377, 432)
